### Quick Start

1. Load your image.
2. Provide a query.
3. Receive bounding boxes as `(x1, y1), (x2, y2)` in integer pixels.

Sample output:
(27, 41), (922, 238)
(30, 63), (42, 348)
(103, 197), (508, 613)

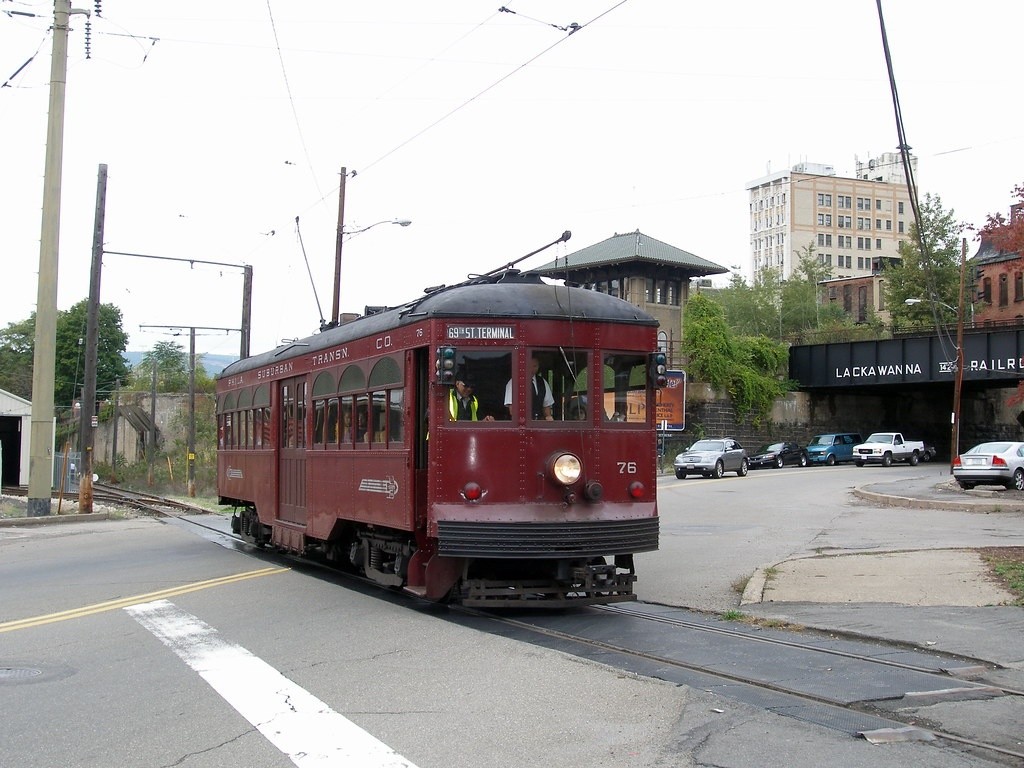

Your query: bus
(214, 263), (667, 615)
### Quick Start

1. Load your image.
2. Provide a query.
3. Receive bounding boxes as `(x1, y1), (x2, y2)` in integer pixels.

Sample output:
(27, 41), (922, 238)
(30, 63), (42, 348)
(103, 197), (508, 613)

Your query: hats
(456, 369), (478, 386)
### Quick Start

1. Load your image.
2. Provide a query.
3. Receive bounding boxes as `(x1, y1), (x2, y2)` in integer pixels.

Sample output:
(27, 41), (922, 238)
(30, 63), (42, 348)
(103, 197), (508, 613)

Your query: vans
(806, 433), (867, 466)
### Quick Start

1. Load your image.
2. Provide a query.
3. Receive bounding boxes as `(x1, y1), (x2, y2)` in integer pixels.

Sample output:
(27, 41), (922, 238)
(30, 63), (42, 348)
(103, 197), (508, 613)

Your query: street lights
(341, 218), (411, 235)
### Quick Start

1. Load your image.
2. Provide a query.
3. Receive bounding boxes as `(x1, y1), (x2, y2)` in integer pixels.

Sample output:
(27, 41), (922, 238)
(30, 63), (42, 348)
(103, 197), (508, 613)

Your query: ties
(532, 379), (536, 396)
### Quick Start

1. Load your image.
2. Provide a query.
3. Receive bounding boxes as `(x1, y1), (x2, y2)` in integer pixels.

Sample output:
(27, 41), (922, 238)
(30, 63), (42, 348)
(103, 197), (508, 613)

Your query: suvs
(674, 436), (750, 480)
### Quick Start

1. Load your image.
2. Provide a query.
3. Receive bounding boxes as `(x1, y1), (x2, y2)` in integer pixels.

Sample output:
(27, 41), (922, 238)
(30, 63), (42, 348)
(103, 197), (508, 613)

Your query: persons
(657, 439), (667, 470)
(220, 396), (406, 450)
(424, 367), (495, 440)
(504, 356), (555, 421)
(570, 405), (586, 421)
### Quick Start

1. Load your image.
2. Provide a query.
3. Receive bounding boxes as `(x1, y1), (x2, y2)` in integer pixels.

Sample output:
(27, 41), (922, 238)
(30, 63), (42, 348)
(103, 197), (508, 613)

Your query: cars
(918, 443), (936, 462)
(953, 442), (1024, 490)
(746, 442), (811, 468)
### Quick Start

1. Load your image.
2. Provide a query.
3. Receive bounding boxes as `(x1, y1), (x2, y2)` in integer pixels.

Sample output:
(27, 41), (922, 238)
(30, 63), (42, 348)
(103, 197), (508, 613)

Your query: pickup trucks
(852, 433), (925, 467)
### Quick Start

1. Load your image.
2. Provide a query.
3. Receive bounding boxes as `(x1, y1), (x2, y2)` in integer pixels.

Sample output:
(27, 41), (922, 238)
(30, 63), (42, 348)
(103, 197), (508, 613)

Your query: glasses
(460, 381), (476, 388)
